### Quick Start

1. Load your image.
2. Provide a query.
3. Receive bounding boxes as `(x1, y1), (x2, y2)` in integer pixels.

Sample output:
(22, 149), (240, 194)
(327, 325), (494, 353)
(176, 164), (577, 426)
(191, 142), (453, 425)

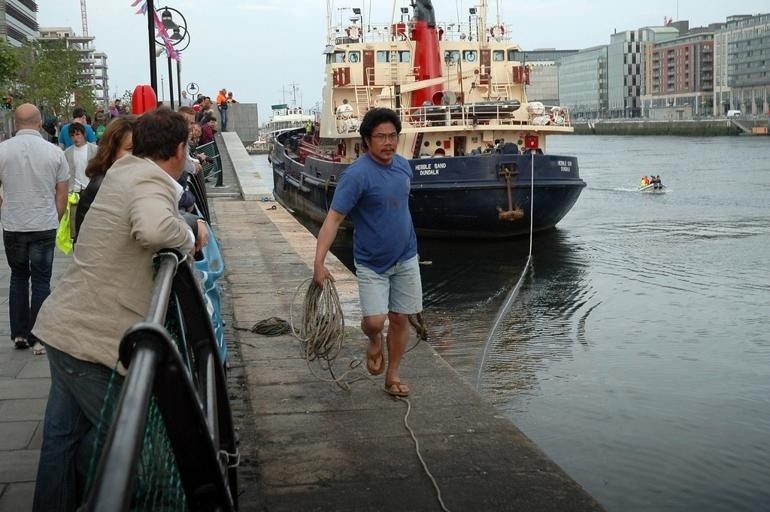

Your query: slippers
(384, 381), (410, 396)
(366, 332), (385, 376)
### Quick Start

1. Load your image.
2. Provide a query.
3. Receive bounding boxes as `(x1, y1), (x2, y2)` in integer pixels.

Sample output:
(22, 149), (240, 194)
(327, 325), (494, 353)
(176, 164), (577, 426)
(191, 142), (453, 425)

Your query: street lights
(153, 7), (199, 113)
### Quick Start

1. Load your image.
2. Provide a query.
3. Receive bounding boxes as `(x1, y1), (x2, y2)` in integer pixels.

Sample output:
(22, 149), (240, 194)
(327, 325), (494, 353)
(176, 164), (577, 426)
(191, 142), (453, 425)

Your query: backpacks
(95, 124), (105, 139)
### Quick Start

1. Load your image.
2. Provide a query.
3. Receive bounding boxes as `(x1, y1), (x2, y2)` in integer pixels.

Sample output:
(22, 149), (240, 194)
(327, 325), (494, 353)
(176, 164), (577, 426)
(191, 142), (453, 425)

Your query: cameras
(200, 152), (214, 163)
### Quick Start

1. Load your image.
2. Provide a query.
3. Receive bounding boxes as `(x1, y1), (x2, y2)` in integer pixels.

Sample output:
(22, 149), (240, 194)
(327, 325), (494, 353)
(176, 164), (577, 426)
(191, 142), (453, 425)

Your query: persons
(640, 175), (663, 190)
(439, 26), (444, 41)
(485, 138), (505, 153)
(30, 108), (201, 512)
(310, 106), (427, 398)
(305, 119), (313, 134)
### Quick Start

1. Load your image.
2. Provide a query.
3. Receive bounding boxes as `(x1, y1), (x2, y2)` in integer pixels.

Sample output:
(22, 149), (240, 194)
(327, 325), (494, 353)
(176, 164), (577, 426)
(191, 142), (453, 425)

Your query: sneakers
(14, 336), (47, 355)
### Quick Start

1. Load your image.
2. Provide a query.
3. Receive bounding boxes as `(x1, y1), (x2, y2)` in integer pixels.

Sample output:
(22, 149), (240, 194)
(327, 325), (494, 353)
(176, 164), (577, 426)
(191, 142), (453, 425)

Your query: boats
(641, 185), (666, 193)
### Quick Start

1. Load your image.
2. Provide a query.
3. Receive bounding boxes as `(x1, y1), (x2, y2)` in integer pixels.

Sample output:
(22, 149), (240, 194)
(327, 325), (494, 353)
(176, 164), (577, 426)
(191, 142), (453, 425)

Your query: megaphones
(154, 45), (164, 58)
